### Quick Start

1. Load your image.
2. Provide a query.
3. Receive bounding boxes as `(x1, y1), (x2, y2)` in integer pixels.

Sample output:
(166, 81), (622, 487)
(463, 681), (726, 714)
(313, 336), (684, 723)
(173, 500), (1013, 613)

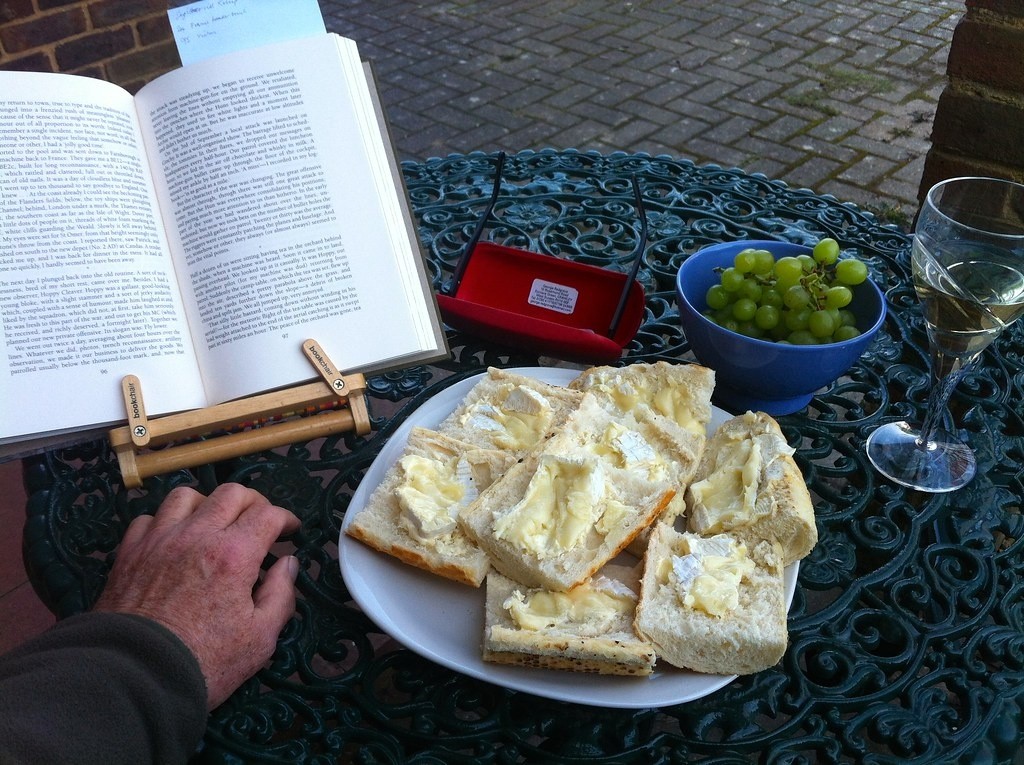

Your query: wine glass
(866, 176), (1024, 493)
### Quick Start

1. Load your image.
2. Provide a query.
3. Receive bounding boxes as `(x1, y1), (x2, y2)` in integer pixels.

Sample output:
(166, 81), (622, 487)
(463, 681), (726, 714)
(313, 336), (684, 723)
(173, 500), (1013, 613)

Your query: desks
(18, 147), (1024, 765)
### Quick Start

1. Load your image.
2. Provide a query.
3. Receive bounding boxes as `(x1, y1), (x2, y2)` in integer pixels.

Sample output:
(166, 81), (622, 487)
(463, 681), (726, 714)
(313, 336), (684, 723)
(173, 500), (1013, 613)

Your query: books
(0, 32), (453, 465)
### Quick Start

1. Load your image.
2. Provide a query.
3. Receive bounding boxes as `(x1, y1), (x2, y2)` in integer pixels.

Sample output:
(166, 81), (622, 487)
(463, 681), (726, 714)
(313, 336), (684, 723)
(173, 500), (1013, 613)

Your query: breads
(344, 361), (818, 676)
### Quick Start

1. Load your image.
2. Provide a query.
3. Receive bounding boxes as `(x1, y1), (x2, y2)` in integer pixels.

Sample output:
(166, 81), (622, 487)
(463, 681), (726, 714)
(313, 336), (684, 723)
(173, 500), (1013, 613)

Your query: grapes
(700, 238), (868, 347)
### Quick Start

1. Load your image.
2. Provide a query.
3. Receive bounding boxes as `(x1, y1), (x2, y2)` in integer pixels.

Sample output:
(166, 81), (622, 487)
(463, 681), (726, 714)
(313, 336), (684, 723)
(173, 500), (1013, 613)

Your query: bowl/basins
(676, 240), (887, 417)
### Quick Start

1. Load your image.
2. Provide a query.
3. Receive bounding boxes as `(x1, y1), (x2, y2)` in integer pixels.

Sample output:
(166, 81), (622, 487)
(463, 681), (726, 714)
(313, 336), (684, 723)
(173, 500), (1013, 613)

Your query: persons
(0, 483), (303, 765)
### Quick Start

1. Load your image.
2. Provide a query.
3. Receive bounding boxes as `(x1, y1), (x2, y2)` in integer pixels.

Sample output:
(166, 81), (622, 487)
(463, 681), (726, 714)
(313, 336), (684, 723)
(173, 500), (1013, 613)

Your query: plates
(339, 365), (801, 708)
